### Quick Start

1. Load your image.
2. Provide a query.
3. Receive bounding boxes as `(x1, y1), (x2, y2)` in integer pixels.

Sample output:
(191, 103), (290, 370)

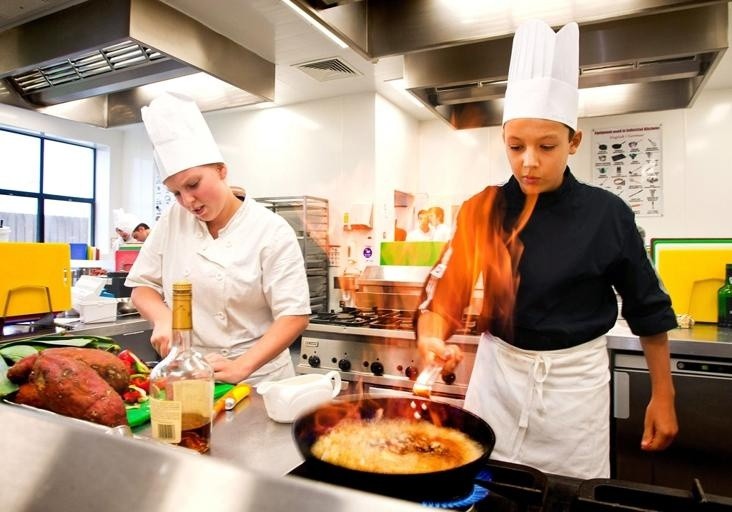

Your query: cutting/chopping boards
(1, 240), (73, 318)
(122, 363), (238, 428)
(656, 247), (732, 326)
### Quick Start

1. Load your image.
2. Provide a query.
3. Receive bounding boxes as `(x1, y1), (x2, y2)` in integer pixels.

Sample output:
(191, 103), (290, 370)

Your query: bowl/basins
(116, 296), (142, 317)
(362, 264), (385, 279)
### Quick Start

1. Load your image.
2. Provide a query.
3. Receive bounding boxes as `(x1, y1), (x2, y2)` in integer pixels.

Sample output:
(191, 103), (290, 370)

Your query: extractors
(397, 0), (731, 136)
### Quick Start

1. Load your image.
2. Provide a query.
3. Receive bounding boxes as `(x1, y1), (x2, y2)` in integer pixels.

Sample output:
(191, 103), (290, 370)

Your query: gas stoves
(286, 450), (732, 512)
(309, 305), (484, 336)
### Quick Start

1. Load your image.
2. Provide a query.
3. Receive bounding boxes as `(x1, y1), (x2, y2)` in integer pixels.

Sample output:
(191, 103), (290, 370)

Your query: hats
(112, 208), (142, 237)
(140, 92), (224, 184)
(502, 20), (579, 130)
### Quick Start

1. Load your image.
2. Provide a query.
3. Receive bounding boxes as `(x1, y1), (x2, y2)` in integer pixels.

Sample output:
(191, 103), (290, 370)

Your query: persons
(94, 224), (131, 275)
(409, 116), (682, 482)
(121, 160), (314, 385)
(131, 222), (151, 242)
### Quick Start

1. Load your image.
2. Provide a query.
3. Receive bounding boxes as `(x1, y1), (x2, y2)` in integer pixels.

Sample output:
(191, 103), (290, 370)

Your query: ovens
(610, 353), (731, 492)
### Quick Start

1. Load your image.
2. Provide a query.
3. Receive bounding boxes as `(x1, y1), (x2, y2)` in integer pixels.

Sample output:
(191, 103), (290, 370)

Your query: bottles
(341, 257), (361, 307)
(717, 263), (732, 329)
(148, 282), (214, 457)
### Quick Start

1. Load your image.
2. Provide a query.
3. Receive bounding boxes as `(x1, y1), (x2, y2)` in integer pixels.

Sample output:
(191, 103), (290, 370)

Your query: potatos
(5, 346), (130, 428)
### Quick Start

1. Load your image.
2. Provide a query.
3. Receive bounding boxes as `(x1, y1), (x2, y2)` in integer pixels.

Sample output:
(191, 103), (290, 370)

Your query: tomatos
(116, 350), (151, 403)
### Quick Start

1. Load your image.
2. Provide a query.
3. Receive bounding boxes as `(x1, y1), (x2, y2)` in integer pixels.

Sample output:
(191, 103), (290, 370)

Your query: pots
(291, 353), (498, 498)
(597, 139), (641, 150)
